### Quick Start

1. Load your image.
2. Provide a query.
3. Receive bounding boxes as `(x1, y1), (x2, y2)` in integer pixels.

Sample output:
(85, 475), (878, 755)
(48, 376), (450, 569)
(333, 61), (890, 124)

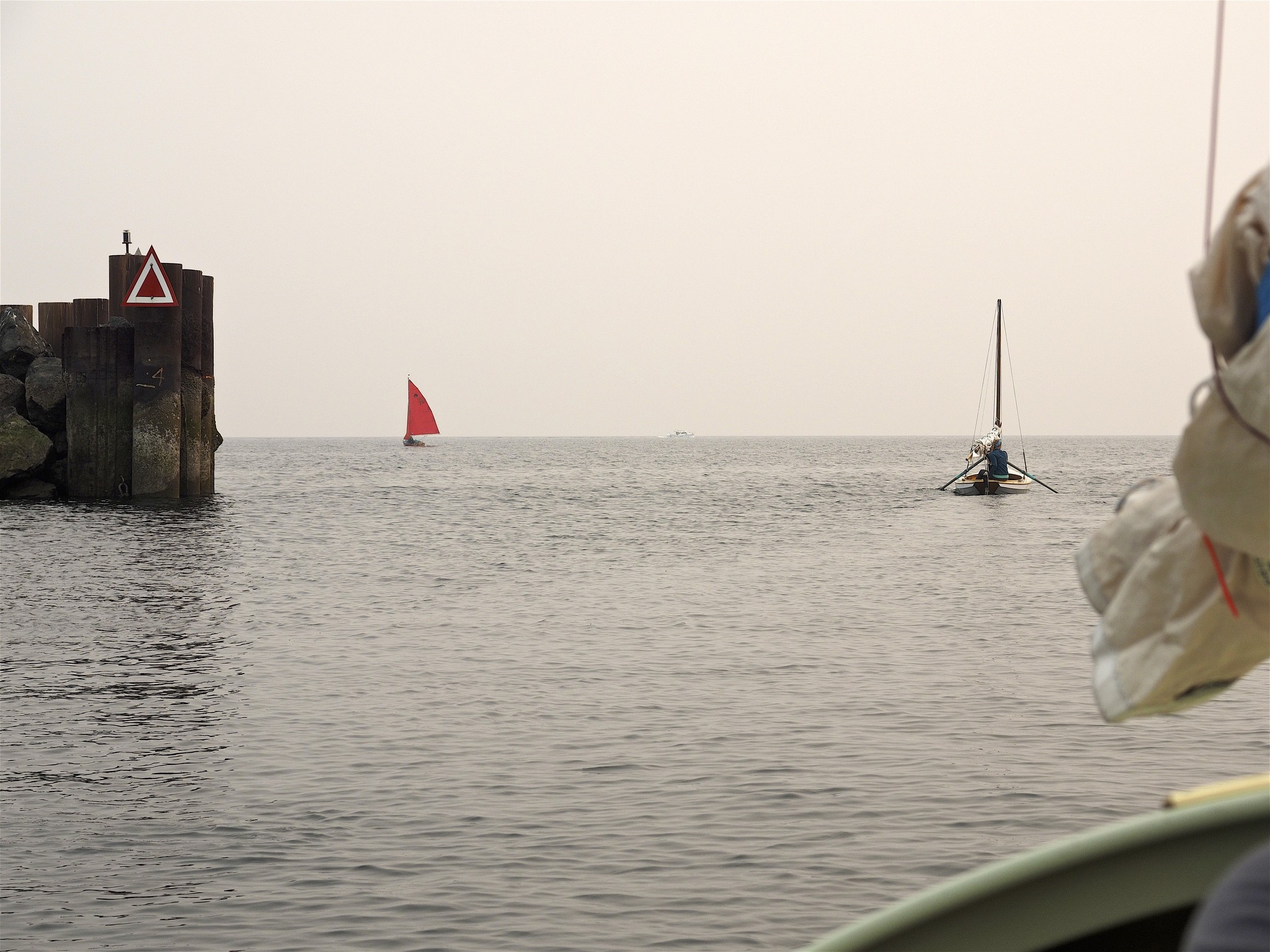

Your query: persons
(1179, 846), (1270, 952)
(407, 435), (415, 444)
(975, 439), (1009, 480)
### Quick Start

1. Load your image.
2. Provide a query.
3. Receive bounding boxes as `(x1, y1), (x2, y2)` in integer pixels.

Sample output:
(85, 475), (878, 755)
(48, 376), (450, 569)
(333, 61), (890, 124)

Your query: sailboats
(402, 374), (441, 446)
(938, 299), (1059, 497)
